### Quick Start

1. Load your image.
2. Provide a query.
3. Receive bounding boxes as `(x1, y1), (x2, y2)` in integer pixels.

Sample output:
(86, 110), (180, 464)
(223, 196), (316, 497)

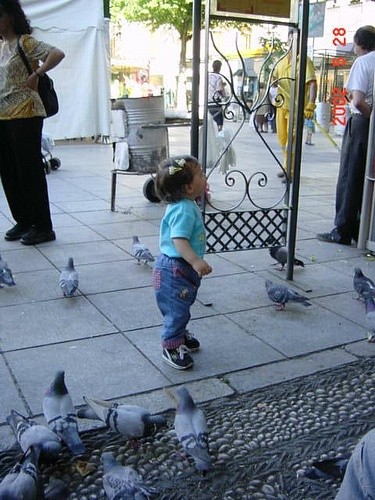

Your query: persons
(208, 60), (226, 131)
(253, 81), (277, 133)
(152, 154), (211, 368)
(272, 29), (317, 183)
(304, 111), (316, 145)
(335, 429), (375, 500)
(0, 0), (65, 246)
(317, 25), (375, 245)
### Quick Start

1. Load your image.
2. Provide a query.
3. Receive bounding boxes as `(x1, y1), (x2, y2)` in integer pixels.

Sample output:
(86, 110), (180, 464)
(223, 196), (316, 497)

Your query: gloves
(303, 102), (316, 120)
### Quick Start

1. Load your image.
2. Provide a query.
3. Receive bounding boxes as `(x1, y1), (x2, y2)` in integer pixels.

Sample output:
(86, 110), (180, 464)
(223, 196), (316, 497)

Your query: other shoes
(277, 172), (284, 177)
(306, 141), (313, 145)
(281, 179), (288, 183)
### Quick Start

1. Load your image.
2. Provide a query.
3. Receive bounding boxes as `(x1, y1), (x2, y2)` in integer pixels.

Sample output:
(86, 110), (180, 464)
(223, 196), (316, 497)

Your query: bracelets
(35, 70), (41, 77)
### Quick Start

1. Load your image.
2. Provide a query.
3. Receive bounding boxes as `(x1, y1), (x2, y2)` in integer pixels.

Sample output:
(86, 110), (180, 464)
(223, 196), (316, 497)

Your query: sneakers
(163, 344), (194, 369)
(317, 231), (334, 242)
(183, 330), (200, 351)
(20, 225), (55, 245)
(4, 223), (30, 241)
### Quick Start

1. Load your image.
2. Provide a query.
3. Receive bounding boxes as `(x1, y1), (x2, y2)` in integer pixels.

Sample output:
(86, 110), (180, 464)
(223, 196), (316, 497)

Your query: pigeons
(174, 392), (213, 470)
(42, 369), (86, 454)
(0, 255), (17, 288)
(353, 267), (375, 300)
(265, 278), (313, 311)
(0, 445), (46, 500)
(7, 409), (61, 465)
(132, 235), (157, 265)
(58, 257), (79, 299)
(74, 395), (167, 451)
(364, 298), (375, 342)
(269, 246), (304, 271)
(98, 452), (160, 500)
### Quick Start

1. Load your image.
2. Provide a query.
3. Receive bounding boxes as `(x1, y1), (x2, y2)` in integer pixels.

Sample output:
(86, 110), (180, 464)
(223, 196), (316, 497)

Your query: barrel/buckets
(110, 92), (171, 174)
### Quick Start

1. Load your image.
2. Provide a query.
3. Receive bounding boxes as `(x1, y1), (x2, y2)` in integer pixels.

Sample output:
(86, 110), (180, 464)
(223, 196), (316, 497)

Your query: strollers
(41, 135), (61, 174)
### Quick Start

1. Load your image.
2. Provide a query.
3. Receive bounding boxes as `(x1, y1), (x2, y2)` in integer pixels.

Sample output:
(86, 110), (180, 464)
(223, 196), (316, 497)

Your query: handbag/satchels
(35, 73), (58, 119)
